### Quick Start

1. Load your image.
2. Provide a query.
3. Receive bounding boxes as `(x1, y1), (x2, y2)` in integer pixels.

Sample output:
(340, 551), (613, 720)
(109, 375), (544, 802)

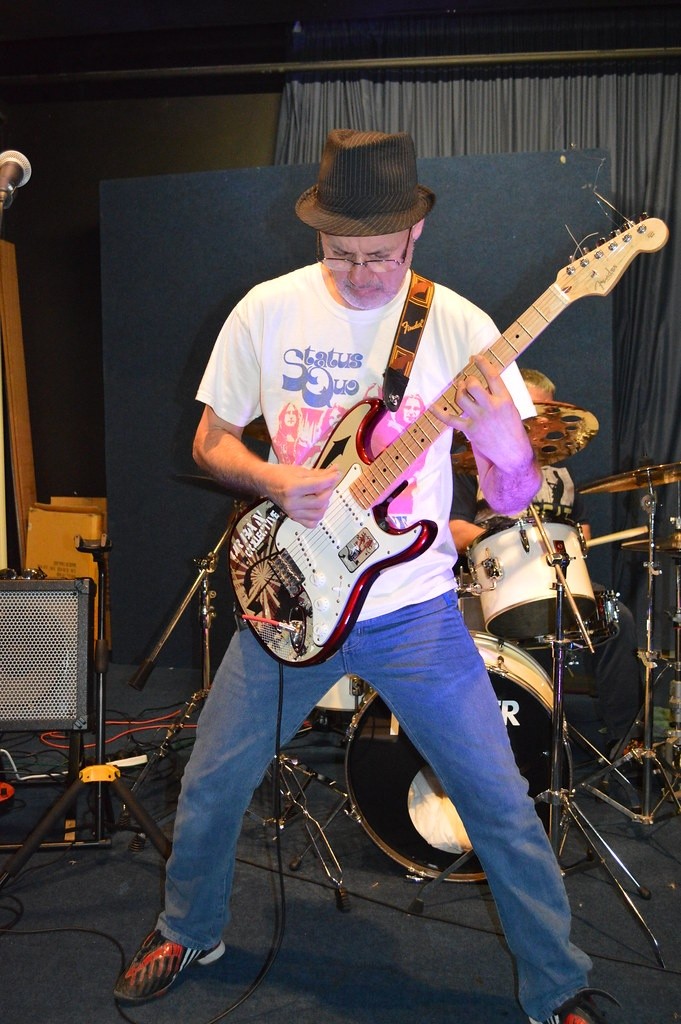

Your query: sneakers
(114, 930), (226, 1002)
(530, 989), (623, 1024)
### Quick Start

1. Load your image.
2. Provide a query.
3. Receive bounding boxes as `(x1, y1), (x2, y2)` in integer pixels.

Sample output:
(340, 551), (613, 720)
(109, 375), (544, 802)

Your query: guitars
(224, 189), (669, 672)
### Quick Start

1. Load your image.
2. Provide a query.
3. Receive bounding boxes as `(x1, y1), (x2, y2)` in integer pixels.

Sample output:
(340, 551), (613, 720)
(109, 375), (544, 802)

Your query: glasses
(315, 230), (412, 274)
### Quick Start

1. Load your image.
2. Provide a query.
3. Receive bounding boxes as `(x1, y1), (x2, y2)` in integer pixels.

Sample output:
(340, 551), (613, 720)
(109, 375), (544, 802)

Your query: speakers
(0, 577), (97, 732)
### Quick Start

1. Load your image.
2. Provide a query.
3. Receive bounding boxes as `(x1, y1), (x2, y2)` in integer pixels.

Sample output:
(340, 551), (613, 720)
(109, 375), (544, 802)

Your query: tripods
(116, 496), (351, 916)
(410, 481), (681, 971)
(0, 532), (173, 891)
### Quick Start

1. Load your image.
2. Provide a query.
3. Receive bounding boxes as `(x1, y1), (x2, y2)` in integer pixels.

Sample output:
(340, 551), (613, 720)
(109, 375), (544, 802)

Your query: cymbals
(170, 473), (257, 502)
(449, 398), (601, 477)
(242, 419), (272, 444)
(574, 461), (681, 495)
(621, 533), (681, 555)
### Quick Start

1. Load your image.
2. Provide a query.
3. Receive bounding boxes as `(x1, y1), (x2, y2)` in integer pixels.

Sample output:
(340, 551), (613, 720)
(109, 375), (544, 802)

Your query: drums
(465, 515), (598, 645)
(341, 627), (568, 884)
(517, 589), (622, 652)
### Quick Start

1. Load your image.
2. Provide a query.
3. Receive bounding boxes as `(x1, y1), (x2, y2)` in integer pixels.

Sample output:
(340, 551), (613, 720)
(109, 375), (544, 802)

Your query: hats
(295, 129), (436, 236)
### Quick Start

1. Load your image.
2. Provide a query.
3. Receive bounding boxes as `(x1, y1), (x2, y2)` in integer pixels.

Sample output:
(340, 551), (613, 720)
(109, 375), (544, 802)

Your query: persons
(111, 126), (624, 1024)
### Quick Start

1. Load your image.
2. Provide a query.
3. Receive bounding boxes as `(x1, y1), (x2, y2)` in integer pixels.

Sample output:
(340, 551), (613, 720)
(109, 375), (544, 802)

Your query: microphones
(0, 150), (32, 208)
